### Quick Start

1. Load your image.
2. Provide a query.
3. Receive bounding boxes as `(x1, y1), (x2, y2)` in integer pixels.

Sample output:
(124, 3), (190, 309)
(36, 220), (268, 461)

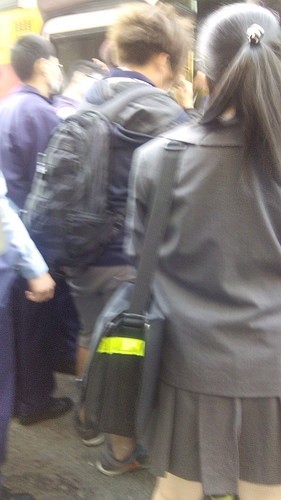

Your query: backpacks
(16, 86), (181, 255)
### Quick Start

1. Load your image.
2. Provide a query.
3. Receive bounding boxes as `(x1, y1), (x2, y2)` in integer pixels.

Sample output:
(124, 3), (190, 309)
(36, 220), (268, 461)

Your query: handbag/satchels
(73, 281), (167, 440)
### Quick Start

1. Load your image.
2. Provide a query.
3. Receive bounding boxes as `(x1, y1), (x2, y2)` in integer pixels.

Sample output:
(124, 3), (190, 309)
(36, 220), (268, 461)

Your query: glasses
(53, 62), (66, 71)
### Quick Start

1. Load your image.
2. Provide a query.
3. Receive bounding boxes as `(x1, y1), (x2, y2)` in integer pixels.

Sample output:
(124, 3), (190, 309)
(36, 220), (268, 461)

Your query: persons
(58, 3), (205, 477)
(128, 4), (281, 500)
(0, 34), (74, 426)
(0, 176), (56, 500)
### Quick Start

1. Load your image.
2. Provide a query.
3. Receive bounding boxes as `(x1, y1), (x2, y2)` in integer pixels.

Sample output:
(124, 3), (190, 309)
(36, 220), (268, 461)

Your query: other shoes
(73, 413), (105, 446)
(98, 451), (149, 476)
(19, 397), (73, 426)
